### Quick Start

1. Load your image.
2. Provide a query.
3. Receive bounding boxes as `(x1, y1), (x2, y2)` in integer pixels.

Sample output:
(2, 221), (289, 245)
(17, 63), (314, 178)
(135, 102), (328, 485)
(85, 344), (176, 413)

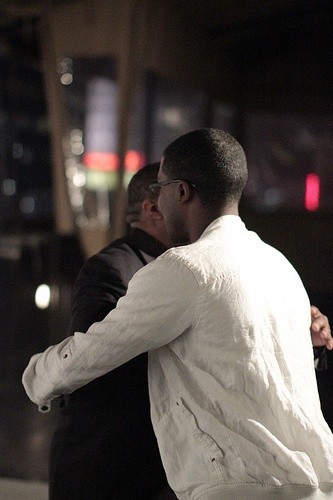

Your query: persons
(46, 157), (333, 500)
(20, 125), (332, 500)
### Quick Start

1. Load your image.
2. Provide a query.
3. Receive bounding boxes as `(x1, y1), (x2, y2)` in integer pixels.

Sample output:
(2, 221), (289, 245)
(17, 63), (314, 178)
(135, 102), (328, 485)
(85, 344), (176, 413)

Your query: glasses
(148, 180), (196, 195)
